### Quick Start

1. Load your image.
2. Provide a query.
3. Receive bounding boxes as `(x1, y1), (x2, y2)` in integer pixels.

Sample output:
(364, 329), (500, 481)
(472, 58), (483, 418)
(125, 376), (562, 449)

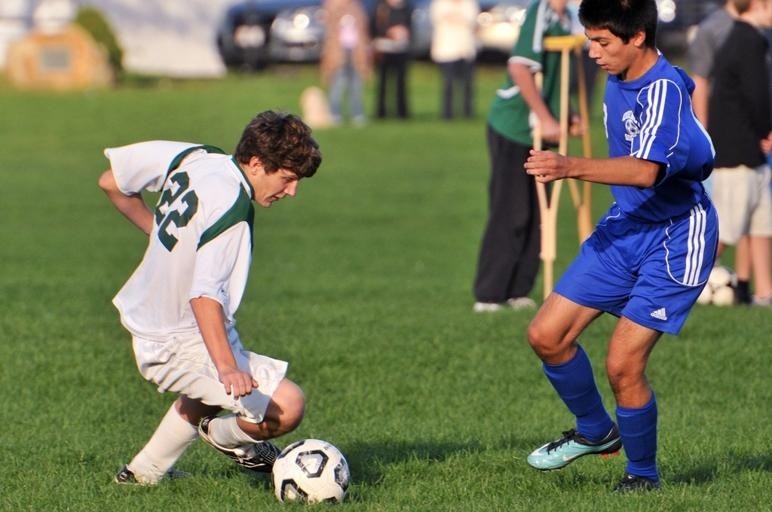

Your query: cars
(212, 0), (717, 71)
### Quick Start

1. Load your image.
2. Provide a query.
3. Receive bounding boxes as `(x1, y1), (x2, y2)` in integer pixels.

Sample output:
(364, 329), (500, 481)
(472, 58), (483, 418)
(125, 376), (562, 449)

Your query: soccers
(695, 266), (738, 306)
(271, 439), (350, 505)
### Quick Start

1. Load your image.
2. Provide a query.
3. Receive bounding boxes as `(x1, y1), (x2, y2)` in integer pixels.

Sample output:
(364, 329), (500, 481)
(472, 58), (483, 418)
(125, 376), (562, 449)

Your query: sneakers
(527, 423), (622, 471)
(198, 414), (282, 473)
(114, 464), (195, 486)
(610, 475), (660, 494)
(473, 297), (535, 315)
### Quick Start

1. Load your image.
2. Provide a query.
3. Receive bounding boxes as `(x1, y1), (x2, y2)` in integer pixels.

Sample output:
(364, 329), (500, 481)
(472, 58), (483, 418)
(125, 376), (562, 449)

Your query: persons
(557, 1), (598, 121)
(318, 0), (371, 130)
(682, 0), (754, 309)
(424, 0), (481, 123)
(522, 0), (721, 493)
(370, 0), (416, 125)
(94, 106), (323, 488)
(468, 0), (586, 314)
(703, 0), (771, 311)
